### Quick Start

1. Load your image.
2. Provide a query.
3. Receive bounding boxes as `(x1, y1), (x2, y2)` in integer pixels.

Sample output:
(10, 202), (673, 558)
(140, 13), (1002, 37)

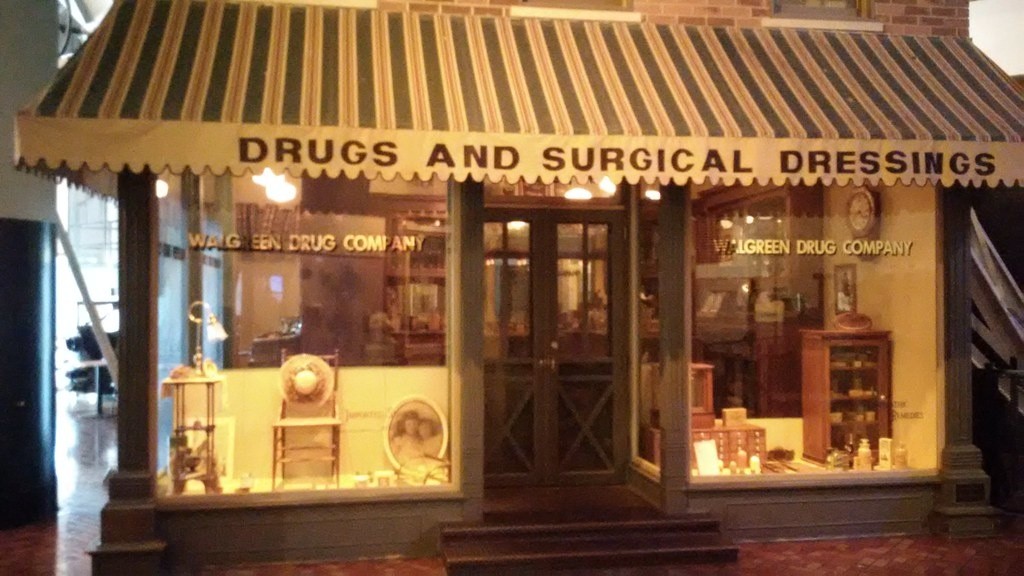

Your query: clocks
(846, 184), (881, 260)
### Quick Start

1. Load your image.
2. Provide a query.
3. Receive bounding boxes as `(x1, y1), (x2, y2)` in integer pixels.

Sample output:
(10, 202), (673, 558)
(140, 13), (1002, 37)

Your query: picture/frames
(833, 263), (858, 316)
(383, 393), (449, 476)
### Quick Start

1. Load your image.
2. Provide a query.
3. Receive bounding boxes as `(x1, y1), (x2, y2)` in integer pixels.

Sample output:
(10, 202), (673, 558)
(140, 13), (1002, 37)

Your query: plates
(385, 397), (449, 471)
(280, 353), (333, 409)
(832, 312), (873, 331)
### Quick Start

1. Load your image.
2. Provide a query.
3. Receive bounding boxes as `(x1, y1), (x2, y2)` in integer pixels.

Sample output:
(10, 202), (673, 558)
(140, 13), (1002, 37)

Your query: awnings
(10, 0), (1024, 207)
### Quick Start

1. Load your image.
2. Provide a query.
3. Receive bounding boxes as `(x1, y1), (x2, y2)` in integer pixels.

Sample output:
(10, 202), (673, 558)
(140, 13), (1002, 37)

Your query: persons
(368, 285), (401, 344)
(556, 301), (582, 330)
(412, 295), (444, 330)
(587, 291), (607, 330)
(393, 410), (443, 465)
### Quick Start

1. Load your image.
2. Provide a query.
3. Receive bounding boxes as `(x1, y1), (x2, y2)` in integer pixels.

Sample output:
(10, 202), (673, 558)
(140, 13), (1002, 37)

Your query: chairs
(270, 348), (344, 491)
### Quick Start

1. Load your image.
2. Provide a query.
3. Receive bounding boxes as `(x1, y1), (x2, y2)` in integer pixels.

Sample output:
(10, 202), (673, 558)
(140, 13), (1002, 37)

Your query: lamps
(719, 211), (735, 230)
(187, 300), (229, 378)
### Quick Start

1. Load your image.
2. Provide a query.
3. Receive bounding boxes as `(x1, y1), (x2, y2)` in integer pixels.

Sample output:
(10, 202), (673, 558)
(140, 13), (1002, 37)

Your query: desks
(163, 375), (225, 494)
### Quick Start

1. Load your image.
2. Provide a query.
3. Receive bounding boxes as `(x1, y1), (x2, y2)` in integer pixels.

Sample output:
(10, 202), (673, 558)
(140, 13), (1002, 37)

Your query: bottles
(858, 437), (907, 470)
(731, 450), (761, 473)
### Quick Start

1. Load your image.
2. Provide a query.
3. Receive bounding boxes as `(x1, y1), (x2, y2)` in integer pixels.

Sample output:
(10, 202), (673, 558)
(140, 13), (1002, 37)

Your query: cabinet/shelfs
(800, 328), (892, 465)
(651, 362), (716, 430)
